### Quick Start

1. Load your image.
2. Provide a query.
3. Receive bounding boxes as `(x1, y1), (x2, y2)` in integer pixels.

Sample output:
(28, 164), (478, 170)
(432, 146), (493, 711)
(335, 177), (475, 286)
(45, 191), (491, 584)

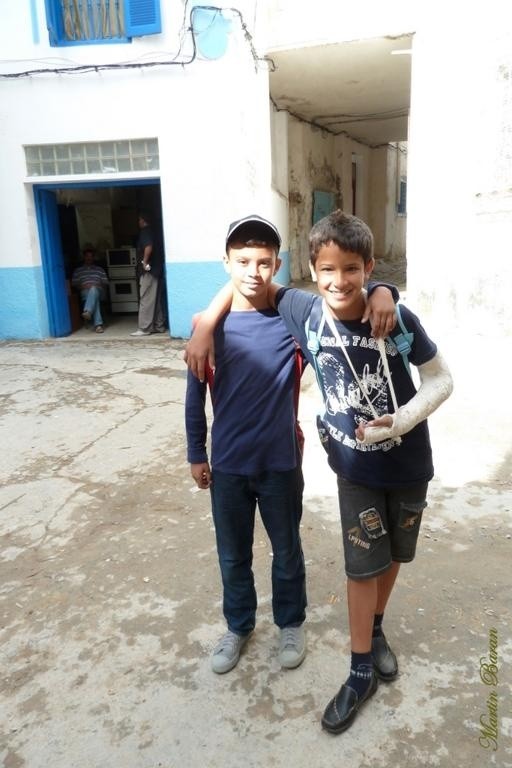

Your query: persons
(71, 249), (110, 333)
(184, 210), (453, 735)
(129, 215), (166, 336)
(185, 214), (400, 675)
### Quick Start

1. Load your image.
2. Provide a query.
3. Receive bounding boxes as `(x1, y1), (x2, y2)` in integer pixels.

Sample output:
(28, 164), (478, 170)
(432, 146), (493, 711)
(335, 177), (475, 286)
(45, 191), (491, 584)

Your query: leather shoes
(370, 624), (399, 681)
(321, 668), (376, 735)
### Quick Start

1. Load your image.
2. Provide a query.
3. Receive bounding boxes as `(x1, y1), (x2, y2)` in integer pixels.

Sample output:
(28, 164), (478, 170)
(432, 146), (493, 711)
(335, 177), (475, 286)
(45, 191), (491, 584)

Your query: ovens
(109, 277), (139, 313)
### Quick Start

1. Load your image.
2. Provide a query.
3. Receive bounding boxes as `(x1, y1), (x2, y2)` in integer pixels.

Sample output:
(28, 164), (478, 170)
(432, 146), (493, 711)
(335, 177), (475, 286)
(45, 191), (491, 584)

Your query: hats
(224, 215), (282, 253)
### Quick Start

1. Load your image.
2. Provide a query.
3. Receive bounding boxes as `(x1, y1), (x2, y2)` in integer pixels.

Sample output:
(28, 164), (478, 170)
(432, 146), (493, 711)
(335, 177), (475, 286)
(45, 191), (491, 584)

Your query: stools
(81, 298), (108, 330)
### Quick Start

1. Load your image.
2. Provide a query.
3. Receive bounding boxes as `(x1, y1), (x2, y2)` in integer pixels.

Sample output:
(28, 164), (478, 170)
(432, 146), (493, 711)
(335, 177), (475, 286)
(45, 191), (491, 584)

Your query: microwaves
(106, 248), (137, 267)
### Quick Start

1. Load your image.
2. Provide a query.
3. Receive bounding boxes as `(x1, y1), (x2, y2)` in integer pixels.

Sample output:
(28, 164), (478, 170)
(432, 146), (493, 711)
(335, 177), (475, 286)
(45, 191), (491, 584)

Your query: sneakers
(95, 325), (105, 333)
(130, 329), (152, 336)
(151, 326), (166, 333)
(82, 310), (91, 320)
(209, 627), (254, 674)
(278, 624), (308, 669)
